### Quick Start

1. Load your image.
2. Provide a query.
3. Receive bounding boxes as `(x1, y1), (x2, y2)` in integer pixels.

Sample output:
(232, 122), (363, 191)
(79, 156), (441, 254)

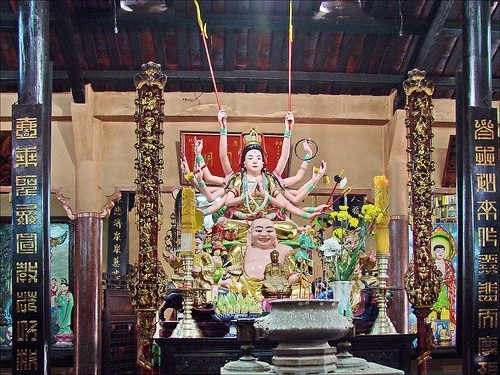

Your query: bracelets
(308, 204), (316, 214)
(283, 130), (292, 138)
(203, 209), (210, 216)
(220, 127), (227, 135)
(198, 180), (206, 188)
(307, 183), (314, 191)
(196, 156), (205, 163)
(303, 154), (312, 163)
(298, 166), (306, 171)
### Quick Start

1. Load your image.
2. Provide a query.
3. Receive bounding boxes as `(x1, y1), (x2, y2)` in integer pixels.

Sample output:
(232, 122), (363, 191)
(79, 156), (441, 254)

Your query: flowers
(318, 204), (384, 282)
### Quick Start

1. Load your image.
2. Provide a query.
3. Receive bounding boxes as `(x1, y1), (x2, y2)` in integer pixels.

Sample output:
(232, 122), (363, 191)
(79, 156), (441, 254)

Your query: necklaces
(242, 167), (270, 215)
(301, 210), (309, 219)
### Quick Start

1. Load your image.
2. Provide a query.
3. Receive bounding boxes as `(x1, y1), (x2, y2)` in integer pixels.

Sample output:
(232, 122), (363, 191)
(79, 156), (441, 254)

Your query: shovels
(307, 187), (351, 224)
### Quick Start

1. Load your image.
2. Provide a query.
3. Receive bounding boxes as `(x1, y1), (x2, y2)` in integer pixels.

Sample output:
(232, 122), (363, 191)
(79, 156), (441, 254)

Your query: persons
(340, 229), (364, 312)
(440, 323), (450, 345)
(54, 277), (74, 335)
(428, 243), (458, 326)
(179, 107), (335, 238)
(51, 277), (58, 305)
(226, 217), (310, 298)
(194, 232), (216, 309)
(259, 248), (293, 297)
(207, 215), (239, 267)
(430, 315), (438, 340)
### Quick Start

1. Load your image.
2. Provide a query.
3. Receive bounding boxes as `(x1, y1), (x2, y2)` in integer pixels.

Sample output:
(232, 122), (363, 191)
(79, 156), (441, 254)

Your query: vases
(329, 280), (356, 318)
